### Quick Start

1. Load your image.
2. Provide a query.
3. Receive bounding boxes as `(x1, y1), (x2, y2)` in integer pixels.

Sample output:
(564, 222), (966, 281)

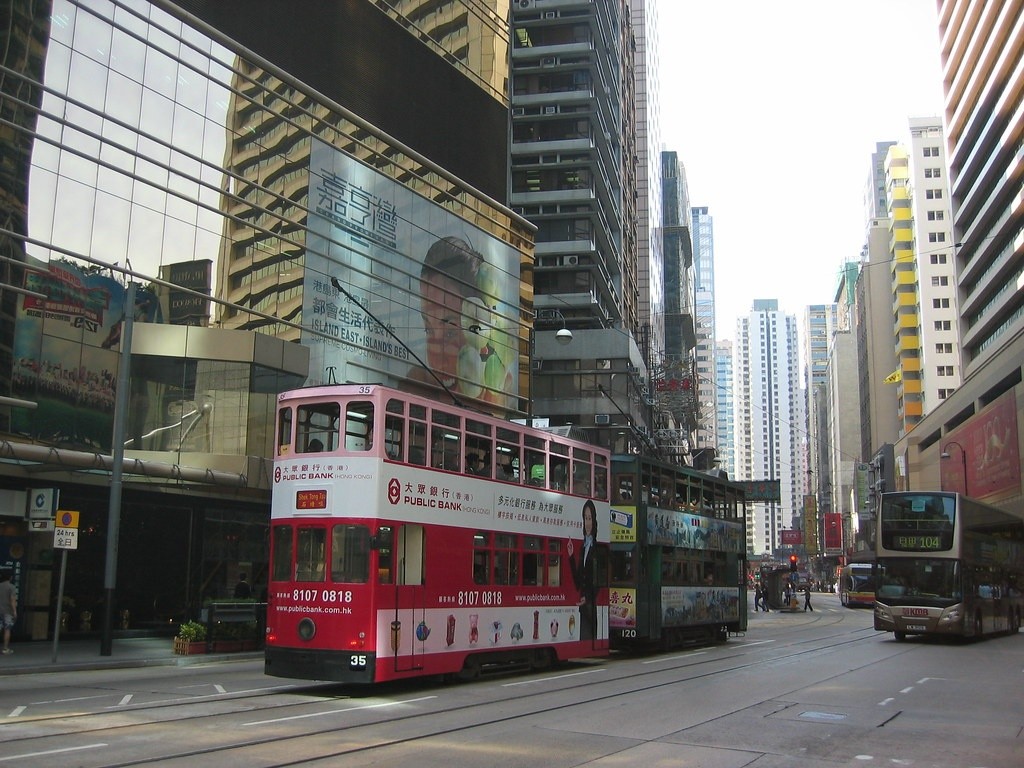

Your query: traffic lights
(791, 554), (796, 567)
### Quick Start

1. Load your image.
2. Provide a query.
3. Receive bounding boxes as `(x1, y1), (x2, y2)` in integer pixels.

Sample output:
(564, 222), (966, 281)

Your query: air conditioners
(546, 107), (556, 114)
(519, 0), (535, 9)
(563, 256), (578, 265)
(633, 216), (639, 224)
(595, 414), (610, 424)
(513, 108), (525, 116)
(629, 139), (634, 146)
(543, 56), (556, 65)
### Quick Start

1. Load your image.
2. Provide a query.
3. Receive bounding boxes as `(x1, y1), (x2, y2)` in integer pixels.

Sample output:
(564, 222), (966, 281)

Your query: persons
(801, 579), (838, 595)
(234, 573), (252, 609)
(566, 500), (606, 642)
(0, 570), (17, 655)
(784, 583), (792, 607)
(753, 584), (763, 612)
(800, 586), (814, 612)
(760, 586), (770, 613)
(652, 487), (715, 515)
(439, 451), (520, 480)
(400, 235), (514, 416)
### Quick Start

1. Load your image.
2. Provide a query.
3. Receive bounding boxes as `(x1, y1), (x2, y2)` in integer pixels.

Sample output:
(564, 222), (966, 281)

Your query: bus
(839, 562), (886, 609)
(873, 490), (1024, 643)
(797, 577), (810, 592)
(264, 382), (748, 686)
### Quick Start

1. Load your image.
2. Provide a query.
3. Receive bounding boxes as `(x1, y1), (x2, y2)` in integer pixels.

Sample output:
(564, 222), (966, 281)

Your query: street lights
(941, 441), (968, 496)
(526, 310), (573, 483)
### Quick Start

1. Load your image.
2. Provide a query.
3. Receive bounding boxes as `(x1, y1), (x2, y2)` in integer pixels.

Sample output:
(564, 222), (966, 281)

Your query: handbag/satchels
(784, 599), (787, 603)
(806, 598), (809, 605)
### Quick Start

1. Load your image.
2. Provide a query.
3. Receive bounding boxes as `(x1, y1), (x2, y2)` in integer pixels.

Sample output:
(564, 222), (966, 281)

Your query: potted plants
(172, 619), (208, 655)
(546, 12), (555, 18)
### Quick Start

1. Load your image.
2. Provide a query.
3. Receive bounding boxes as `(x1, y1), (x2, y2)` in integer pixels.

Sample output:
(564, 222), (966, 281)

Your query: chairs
(396, 446), (424, 465)
(309, 438), (324, 452)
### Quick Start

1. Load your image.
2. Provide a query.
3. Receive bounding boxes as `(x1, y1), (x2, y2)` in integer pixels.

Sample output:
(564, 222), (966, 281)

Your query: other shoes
(2, 648), (14, 654)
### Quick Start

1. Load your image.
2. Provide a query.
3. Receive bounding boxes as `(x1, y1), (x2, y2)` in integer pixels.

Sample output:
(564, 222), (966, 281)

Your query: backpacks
(759, 590), (762, 599)
